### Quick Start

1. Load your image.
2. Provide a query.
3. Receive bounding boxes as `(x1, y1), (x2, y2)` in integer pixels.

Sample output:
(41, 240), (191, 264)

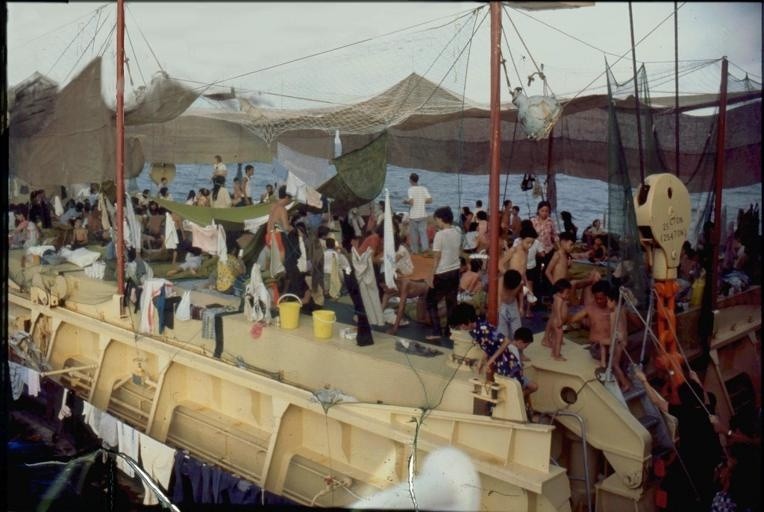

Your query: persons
(7, 154), (764, 512)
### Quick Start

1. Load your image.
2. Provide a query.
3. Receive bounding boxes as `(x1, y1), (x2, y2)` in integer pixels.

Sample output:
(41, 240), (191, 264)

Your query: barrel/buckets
(276, 294), (304, 329)
(312, 310), (336, 340)
